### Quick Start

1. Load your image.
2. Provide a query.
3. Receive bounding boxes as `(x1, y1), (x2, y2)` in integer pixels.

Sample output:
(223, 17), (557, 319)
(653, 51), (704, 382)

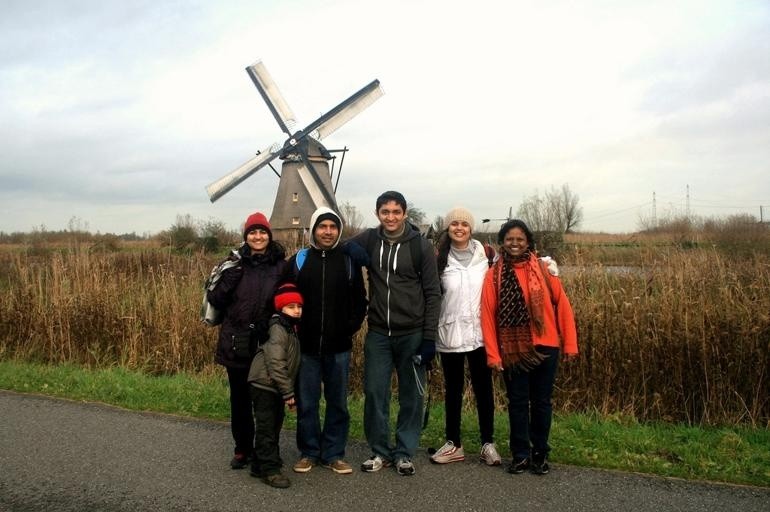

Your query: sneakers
(293, 455), (318, 473)
(394, 458), (415, 475)
(509, 452), (530, 473)
(251, 469), (290, 488)
(231, 449), (255, 469)
(532, 448), (549, 475)
(481, 442), (501, 466)
(430, 440), (465, 464)
(321, 459), (352, 473)
(361, 454), (392, 471)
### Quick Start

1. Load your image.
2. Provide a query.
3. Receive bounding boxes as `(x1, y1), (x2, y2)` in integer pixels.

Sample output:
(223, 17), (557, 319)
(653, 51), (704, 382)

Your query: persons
(428, 206), (561, 467)
(479, 219), (580, 475)
(245, 284), (303, 488)
(207, 212), (292, 470)
(257, 206), (367, 475)
(341, 191), (442, 475)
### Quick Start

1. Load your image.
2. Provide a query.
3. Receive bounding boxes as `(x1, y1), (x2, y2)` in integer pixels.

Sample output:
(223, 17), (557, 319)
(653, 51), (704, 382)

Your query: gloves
(417, 341), (434, 367)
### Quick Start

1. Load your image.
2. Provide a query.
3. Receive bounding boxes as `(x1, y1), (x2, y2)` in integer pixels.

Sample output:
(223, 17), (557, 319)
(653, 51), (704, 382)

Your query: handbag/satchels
(198, 266), (223, 326)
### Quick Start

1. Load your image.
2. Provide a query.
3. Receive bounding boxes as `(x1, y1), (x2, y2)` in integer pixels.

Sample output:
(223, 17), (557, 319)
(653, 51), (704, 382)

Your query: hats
(275, 283), (304, 310)
(244, 212), (272, 241)
(312, 213), (341, 233)
(443, 209), (475, 234)
